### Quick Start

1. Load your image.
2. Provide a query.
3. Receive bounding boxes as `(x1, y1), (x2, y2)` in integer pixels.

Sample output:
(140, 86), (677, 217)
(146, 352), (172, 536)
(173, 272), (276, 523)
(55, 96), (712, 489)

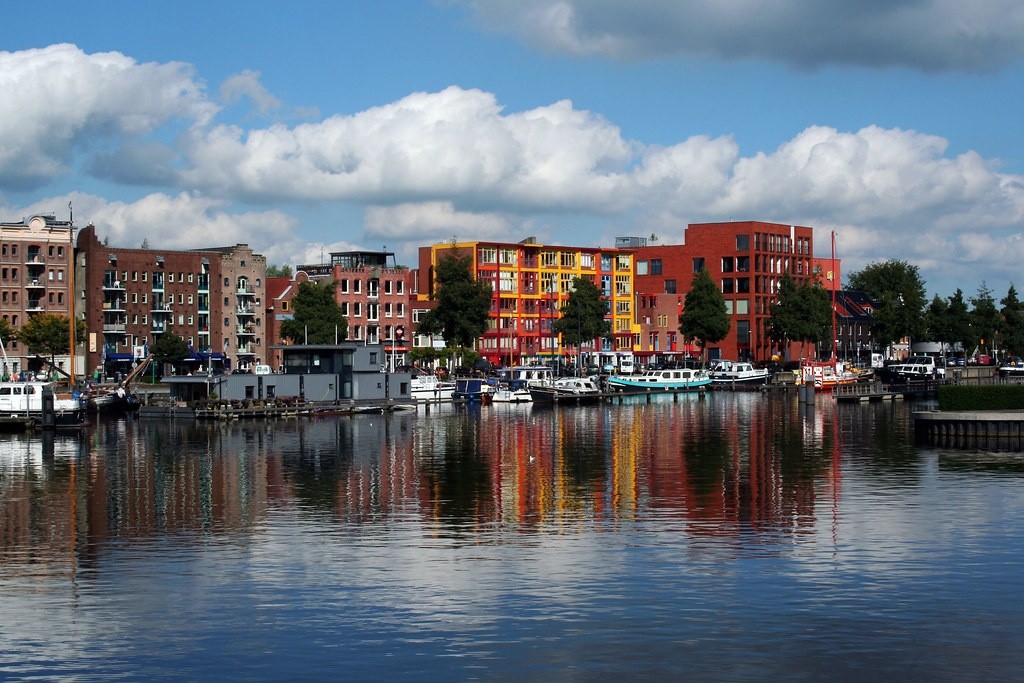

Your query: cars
(957, 358), (966, 366)
(967, 357), (978, 366)
(998, 356), (1023, 366)
(976, 354), (990, 366)
(945, 358), (957, 366)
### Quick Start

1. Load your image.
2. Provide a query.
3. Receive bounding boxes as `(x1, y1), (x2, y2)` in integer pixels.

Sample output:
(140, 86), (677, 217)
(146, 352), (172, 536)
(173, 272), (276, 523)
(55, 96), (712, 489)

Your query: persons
(51, 370), (58, 382)
(2, 370), (36, 382)
(93, 368), (99, 385)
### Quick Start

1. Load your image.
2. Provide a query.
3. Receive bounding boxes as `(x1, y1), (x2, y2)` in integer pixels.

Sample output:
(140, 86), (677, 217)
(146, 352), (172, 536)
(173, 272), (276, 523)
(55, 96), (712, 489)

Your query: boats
(0, 241), (157, 416)
(794, 230), (1024, 391)
(395, 358), (774, 400)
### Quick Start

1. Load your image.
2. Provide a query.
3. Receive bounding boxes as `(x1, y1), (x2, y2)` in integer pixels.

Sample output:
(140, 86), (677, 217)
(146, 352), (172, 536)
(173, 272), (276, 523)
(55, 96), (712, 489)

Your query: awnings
(109, 352), (226, 362)
